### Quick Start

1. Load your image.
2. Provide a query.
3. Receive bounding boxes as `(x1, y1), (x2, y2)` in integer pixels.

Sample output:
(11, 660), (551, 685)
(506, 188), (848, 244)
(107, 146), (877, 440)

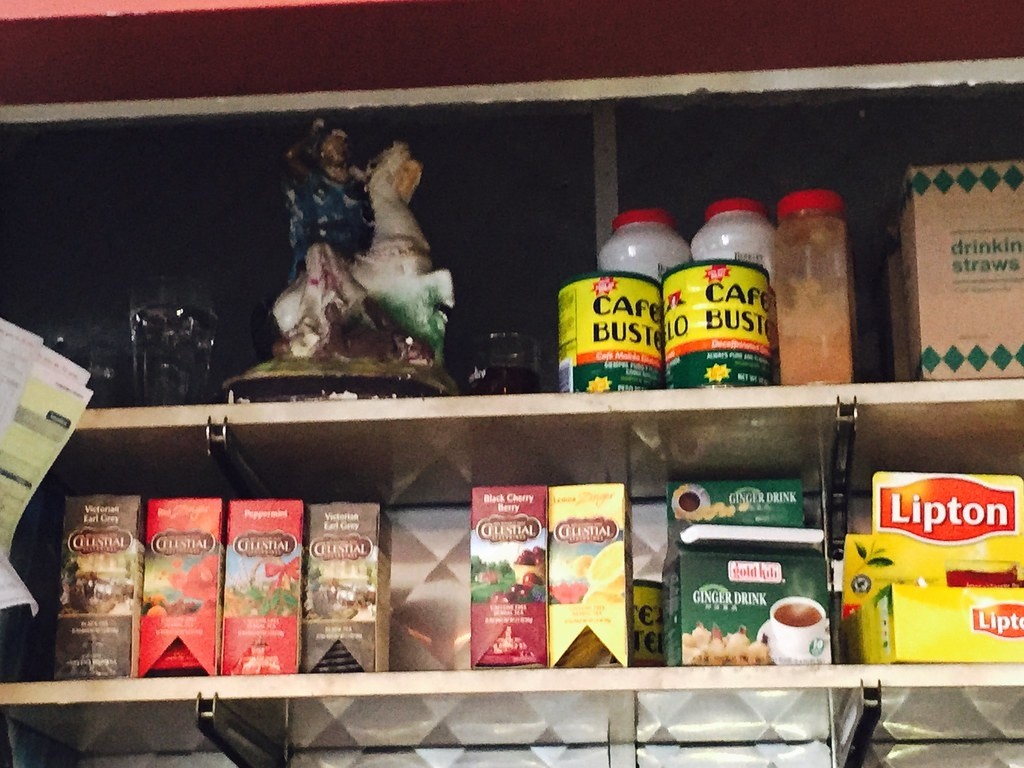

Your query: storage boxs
(662, 478), (832, 667)
(838, 471), (1024, 664)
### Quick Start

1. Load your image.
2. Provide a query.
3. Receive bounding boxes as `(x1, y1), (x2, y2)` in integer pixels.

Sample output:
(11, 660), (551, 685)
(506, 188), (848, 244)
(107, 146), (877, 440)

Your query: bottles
(773, 190), (855, 385)
(689, 199), (775, 276)
(600, 208), (692, 283)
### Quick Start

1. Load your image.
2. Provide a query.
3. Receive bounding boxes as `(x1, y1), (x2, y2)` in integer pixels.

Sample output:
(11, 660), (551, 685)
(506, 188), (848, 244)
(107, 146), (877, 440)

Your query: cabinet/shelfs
(0, 57), (1024, 768)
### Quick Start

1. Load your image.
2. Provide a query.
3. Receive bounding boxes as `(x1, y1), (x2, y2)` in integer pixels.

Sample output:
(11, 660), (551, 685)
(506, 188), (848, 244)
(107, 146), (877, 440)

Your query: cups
(474, 334), (539, 394)
(757, 596), (832, 665)
(130, 304), (222, 406)
